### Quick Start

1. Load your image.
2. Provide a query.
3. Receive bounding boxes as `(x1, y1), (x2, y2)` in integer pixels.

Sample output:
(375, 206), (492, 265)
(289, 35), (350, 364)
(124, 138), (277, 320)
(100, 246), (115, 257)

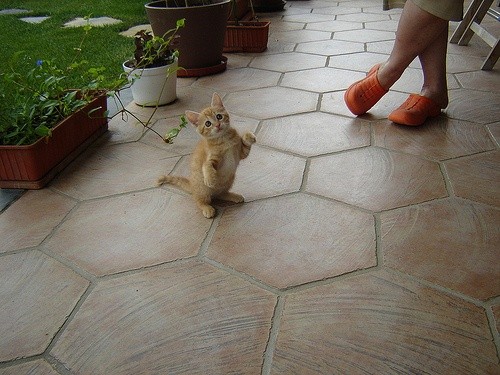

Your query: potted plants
(0, 0), (287, 190)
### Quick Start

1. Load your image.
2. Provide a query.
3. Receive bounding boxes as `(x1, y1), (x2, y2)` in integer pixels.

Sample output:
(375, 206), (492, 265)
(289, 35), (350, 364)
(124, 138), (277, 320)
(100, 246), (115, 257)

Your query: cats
(157, 92), (257, 218)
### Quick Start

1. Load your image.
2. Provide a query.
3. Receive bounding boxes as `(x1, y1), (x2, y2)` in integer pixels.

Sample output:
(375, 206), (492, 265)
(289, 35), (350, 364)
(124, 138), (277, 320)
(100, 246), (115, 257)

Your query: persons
(344, 0), (464, 126)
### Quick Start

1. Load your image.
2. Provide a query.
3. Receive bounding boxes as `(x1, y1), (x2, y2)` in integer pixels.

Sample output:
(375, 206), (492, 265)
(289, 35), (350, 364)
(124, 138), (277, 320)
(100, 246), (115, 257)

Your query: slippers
(344, 64), (388, 115)
(388, 93), (442, 126)
